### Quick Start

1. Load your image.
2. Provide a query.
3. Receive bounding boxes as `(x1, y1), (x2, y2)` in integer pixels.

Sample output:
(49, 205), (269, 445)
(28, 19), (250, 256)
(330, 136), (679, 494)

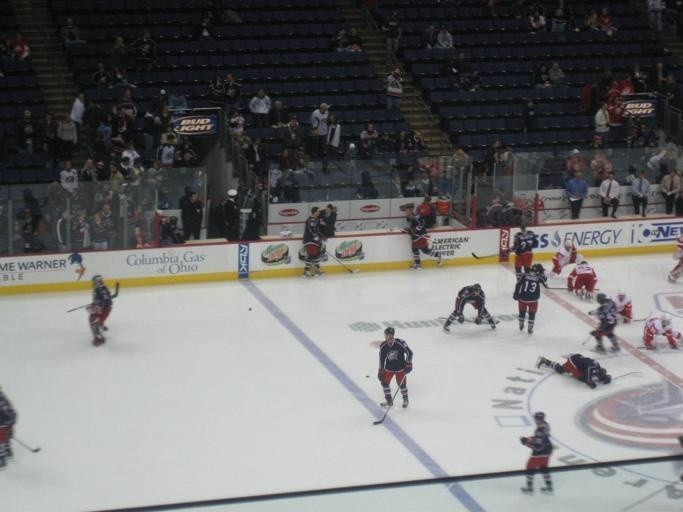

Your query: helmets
(533, 263), (543, 272)
(384, 327), (395, 335)
(661, 314), (671, 322)
(565, 240), (573, 248)
(534, 412), (545, 420)
(597, 294), (605, 301)
(619, 289), (626, 296)
(473, 284), (481, 290)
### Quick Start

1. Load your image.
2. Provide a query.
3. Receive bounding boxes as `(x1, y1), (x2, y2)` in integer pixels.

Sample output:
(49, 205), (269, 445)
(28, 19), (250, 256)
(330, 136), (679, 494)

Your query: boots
(522, 485), (533, 491)
(538, 357), (551, 368)
(541, 481), (552, 490)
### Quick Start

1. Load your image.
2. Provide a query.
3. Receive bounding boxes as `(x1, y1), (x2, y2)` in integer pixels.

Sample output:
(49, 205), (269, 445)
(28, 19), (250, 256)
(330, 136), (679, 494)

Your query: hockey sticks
(631, 310), (652, 324)
(12, 435), (40, 453)
(326, 250), (360, 273)
(610, 370), (642, 382)
(373, 374), (406, 426)
(471, 253), (505, 260)
(441, 320), (500, 324)
(543, 282), (601, 290)
(66, 282), (119, 313)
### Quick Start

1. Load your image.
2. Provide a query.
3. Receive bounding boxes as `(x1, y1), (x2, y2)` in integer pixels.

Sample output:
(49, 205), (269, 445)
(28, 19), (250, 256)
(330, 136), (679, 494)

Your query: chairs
(0, 0), (683, 258)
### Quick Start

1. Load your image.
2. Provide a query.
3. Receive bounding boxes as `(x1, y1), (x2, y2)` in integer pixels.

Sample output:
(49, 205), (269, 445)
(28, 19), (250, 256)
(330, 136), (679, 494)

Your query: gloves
(513, 292), (520, 300)
(475, 317), (481, 324)
(404, 362), (412, 374)
(458, 314), (464, 323)
(378, 369), (384, 380)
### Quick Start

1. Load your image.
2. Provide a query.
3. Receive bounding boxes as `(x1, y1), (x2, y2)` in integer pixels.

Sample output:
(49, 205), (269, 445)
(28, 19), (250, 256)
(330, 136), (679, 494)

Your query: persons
(377, 327), (414, 408)
(642, 316), (683, 352)
(86, 274), (114, 346)
(589, 293), (621, 354)
(0, 1), (683, 332)
(519, 412), (554, 494)
(536, 353), (611, 389)
(0, 389), (17, 468)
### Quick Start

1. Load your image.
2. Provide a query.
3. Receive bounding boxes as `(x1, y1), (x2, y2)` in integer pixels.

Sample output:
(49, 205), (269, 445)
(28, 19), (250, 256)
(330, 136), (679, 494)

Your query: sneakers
(381, 400), (392, 405)
(489, 320), (495, 327)
(443, 323), (449, 331)
(402, 399), (409, 408)
